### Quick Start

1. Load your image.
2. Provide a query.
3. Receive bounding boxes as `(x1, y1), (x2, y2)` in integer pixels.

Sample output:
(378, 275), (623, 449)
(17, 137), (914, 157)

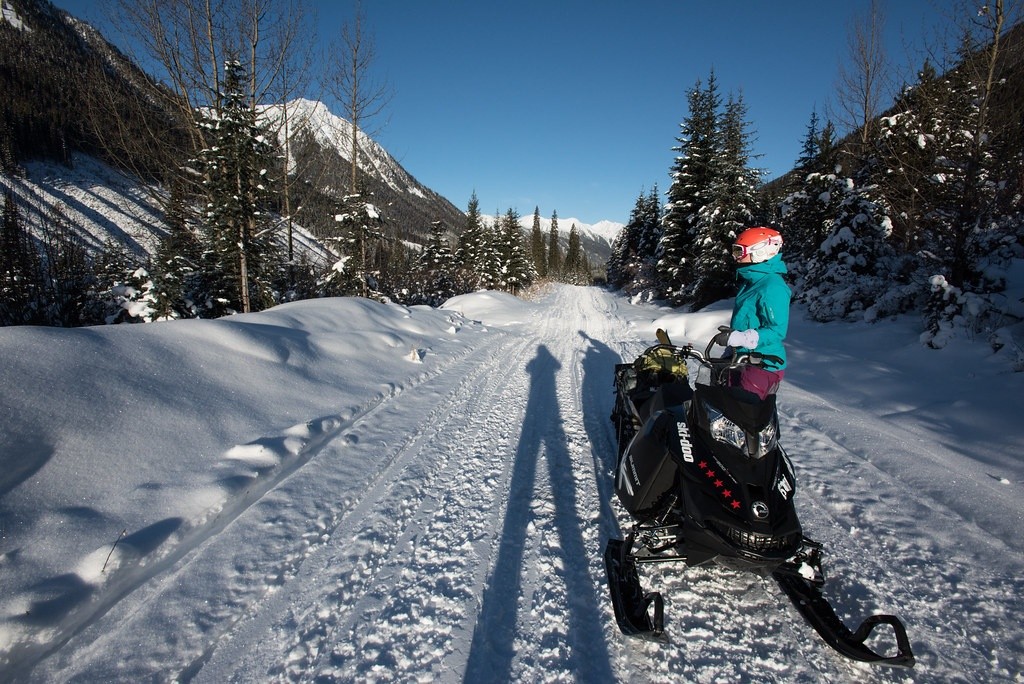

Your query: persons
(716, 225), (793, 397)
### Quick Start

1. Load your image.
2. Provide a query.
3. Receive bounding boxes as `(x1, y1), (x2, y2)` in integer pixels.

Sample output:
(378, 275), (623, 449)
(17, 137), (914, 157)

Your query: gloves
(715, 326), (759, 350)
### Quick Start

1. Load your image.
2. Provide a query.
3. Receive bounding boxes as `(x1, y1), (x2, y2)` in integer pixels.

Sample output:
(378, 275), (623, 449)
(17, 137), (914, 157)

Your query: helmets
(735, 227), (783, 263)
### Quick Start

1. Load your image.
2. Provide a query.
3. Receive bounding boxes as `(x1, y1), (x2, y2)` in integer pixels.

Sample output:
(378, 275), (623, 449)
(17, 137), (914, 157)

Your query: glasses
(731, 244), (746, 261)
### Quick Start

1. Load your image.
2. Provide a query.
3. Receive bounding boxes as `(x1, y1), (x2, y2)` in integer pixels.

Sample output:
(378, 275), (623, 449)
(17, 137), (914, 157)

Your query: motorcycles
(605, 326), (916, 669)
